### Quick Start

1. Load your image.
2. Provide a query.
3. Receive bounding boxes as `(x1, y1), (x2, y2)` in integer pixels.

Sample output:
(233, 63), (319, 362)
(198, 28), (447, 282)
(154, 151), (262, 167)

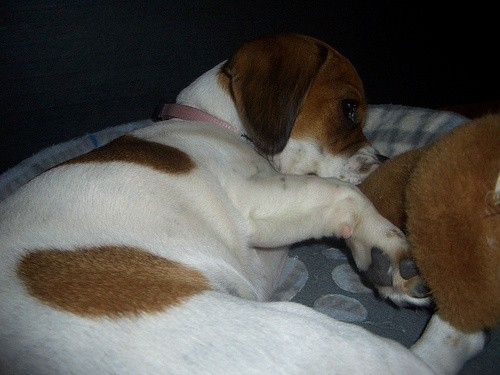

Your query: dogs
(0, 27), (491, 375)
